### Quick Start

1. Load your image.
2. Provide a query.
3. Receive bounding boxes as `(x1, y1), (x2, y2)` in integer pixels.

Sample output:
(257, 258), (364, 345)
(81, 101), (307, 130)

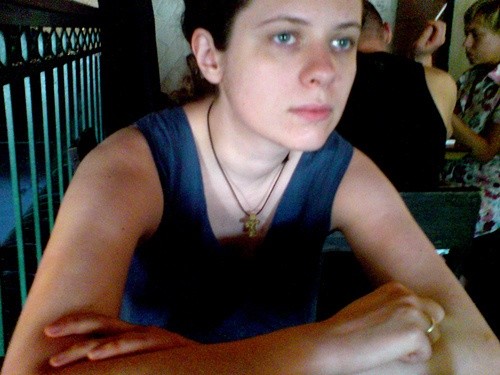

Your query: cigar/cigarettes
(434, 3), (447, 22)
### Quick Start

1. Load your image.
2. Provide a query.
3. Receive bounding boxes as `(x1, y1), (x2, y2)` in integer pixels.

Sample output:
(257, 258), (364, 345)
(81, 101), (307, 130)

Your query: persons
(334, 0), (457, 193)
(0, 0), (500, 375)
(451, 0), (500, 339)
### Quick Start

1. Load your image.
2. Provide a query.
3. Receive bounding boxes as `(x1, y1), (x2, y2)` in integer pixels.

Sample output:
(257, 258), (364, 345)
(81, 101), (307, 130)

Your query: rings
(427, 318), (436, 334)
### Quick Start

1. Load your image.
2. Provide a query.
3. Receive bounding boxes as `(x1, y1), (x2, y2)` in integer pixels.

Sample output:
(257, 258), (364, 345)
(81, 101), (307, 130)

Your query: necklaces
(206, 99), (289, 237)
